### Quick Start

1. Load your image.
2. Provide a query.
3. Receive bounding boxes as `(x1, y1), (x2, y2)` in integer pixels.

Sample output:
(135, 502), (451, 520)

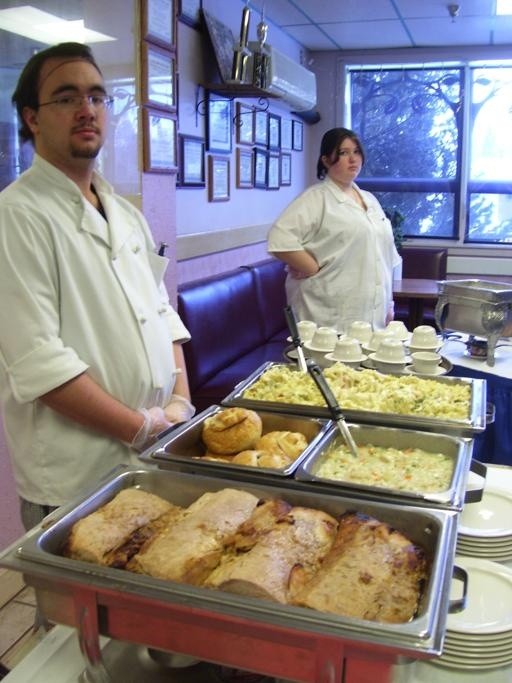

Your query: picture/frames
(268, 155), (280, 190)
(208, 155), (230, 202)
(268, 113), (281, 151)
(141, 0), (178, 52)
(292, 120), (303, 151)
(143, 108), (179, 174)
(279, 153), (292, 186)
(236, 148), (254, 188)
(206, 88), (232, 153)
(236, 102), (256, 145)
(253, 148), (268, 188)
(140, 40), (176, 112)
(253, 105), (269, 146)
(176, 134), (206, 188)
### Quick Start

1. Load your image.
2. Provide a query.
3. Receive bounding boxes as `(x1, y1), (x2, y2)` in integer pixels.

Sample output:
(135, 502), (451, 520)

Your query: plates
(369, 353), (412, 365)
(302, 342), (333, 353)
(362, 341), (378, 353)
(407, 340), (445, 350)
(286, 336), (312, 343)
(407, 356), (453, 378)
(456, 485), (512, 562)
(324, 351), (368, 363)
(429, 556), (512, 672)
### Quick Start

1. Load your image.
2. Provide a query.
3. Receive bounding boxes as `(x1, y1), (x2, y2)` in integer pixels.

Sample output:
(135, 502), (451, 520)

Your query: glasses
(33, 93), (115, 112)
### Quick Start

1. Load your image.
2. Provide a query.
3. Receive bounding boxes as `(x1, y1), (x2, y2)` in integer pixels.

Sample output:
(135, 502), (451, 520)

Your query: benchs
(176, 267), (294, 414)
(243, 245), (448, 343)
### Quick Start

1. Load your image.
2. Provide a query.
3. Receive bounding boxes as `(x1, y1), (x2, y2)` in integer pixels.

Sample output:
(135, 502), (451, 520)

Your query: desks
(388, 279), (440, 332)
(2, 332), (512, 683)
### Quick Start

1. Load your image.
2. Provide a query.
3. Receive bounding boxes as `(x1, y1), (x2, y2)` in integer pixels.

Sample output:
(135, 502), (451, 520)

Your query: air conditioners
(244, 41), (317, 112)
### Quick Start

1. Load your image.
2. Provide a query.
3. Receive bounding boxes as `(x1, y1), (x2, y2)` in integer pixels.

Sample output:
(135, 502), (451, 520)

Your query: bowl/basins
(313, 327), (338, 349)
(413, 352), (441, 374)
(369, 330), (396, 351)
(376, 340), (405, 362)
(297, 321), (317, 342)
(385, 321), (411, 341)
(333, 337), (363, 361)
(349, 320), (372, 342)
(411, 326), (437, 347)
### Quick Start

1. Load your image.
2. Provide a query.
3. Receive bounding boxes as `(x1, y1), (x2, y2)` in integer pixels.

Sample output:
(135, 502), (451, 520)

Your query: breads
(63, 487), (426, 624)
(191, 407), (308, 468)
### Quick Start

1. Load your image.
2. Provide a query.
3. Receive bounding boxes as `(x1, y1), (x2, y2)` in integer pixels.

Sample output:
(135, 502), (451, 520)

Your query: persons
(267, 127), (402, 336)
(0, 43), (195, 532)
